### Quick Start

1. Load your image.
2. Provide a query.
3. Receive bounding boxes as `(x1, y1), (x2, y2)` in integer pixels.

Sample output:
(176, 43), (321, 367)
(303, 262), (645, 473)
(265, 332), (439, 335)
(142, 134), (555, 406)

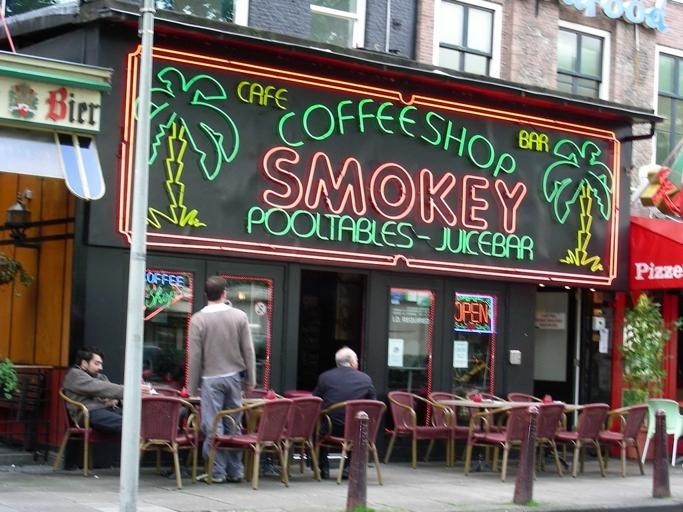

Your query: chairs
(52, 386), (683, 490)
(0, 365), (52, 463)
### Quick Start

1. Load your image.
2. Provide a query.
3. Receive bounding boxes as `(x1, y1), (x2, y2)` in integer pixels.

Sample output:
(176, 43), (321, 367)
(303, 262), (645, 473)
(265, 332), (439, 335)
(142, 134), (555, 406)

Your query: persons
(62, 345), (123, 439)
(184, 276), (256, 483)
(312, 346), (377, 481)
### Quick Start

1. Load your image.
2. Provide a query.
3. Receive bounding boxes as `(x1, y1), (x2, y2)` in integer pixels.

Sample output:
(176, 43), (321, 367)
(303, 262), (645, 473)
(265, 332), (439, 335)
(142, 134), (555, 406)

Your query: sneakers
(194, 472), (229, 483)
(227, 472), (245, 484)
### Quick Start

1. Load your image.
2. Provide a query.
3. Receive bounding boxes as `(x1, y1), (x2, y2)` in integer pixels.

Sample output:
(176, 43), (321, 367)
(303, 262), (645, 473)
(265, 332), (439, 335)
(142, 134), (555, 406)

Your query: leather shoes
(341, 469), (350, 480)
(320, 469), (330, 480)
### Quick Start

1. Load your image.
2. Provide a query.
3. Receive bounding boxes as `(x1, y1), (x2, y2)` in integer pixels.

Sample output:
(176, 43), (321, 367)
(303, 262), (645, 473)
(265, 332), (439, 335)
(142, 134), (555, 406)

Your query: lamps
(5, 192), (41, 250)
